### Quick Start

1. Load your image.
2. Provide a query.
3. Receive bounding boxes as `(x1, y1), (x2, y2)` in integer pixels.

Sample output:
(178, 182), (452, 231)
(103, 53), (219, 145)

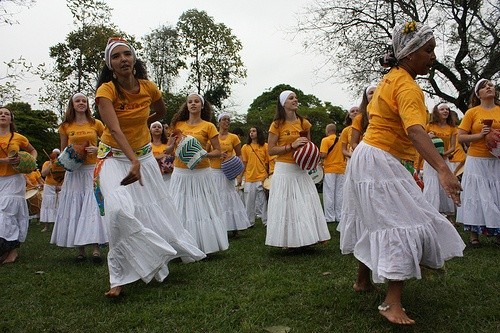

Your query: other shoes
(41, 228), (48, 232)
(37, 222), (42, 226)
(92, 250), (101, 258)
(77, 255), (86, 261)
(482, 229), (489, 236)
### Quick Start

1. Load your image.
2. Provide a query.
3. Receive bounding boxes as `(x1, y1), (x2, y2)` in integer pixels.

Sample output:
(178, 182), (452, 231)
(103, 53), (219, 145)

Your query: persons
(28, 79), (500, 260)
(0, 107), (37, 263)
(95, 38), (207, 296)
(337, 22), (462, 324)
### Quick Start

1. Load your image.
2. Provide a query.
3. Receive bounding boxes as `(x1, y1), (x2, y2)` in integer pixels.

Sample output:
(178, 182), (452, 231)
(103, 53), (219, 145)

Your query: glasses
(222, 117), (231, 122)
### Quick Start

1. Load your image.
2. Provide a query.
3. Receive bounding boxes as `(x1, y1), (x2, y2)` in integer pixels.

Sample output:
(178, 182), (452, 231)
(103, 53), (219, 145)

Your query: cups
(8, 149), (17, 157)
(299, 130), (308, 137)
(484, 119), (493, 127)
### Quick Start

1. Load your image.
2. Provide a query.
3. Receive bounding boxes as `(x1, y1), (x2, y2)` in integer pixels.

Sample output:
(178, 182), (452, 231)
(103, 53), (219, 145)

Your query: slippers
(469, 239), (480, 245)
